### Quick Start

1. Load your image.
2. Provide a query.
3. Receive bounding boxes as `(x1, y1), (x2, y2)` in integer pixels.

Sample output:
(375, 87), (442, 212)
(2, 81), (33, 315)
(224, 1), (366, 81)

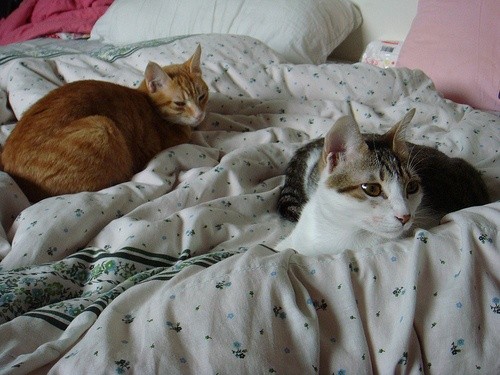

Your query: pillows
(87, 0), (362, 64)
(395, 0), (500, 114)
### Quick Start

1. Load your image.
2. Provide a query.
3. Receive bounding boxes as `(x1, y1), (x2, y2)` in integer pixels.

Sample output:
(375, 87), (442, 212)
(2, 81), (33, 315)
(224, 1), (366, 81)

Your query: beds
(0, 0), (500, 375)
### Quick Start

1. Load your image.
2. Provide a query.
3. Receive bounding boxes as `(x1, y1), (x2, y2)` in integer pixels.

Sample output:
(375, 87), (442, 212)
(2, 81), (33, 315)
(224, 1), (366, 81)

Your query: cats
(0, 45), (208, 195)
(274, 108), (500, 255)
